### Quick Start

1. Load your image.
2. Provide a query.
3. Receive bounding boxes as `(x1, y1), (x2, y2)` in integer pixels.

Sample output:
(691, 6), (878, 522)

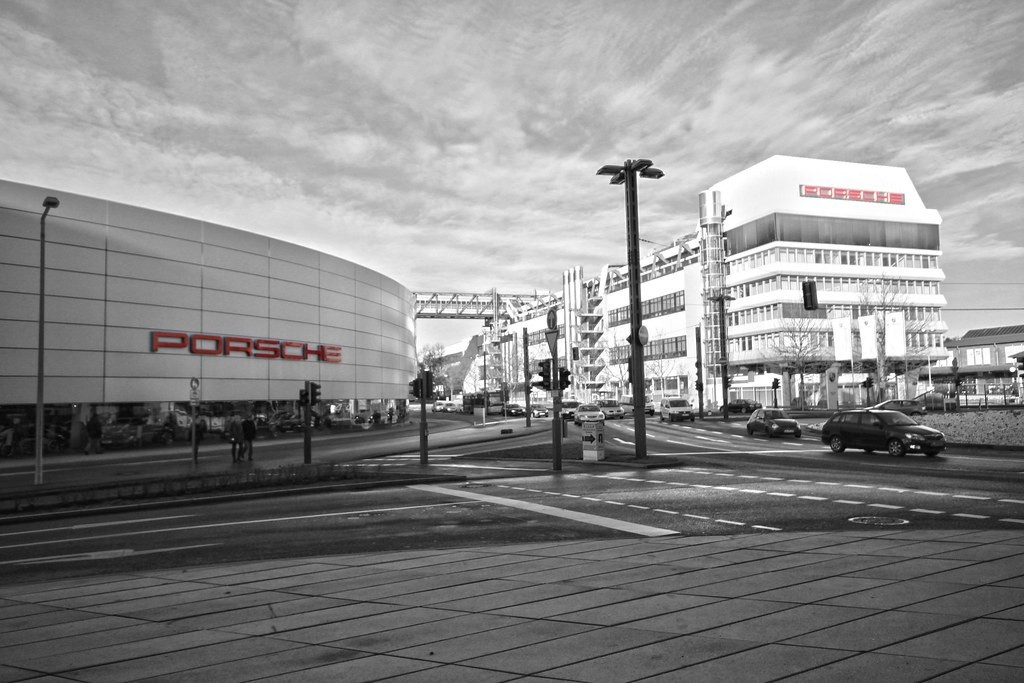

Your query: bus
(463, 391), (503, 414)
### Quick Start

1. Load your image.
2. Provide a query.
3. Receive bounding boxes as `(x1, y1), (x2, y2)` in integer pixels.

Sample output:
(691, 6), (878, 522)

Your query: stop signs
(639, 325), (648, 345)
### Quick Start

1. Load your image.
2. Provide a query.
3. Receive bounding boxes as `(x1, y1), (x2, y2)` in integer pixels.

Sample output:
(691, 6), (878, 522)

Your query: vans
(620, 394), (654, 416)
(660, 398), (695, 423)
(559, 399), (578, 421)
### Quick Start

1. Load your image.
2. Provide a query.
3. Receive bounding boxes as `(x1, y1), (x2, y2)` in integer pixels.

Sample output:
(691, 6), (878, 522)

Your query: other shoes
(248, 458), (253, 460)
(237, 458), (242, 462)
(97, 451), (105, 454)
(233, 460), (237, 463)
(84, 451), (89, 455)
(241, 454), (244, 458)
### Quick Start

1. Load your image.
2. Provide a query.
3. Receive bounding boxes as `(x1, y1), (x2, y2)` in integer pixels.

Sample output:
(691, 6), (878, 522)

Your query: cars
(432, 401), (463, 412)
(596, 400), (624, 418)
(720, 399), (761, 414)
(574, 404), (605, 426)
(502, 404), (527, 417)
(821, 408), (945, 458)
(871, 399), (927, 416)
(747, 408), (802, 438)
(530, 403), (549, 418)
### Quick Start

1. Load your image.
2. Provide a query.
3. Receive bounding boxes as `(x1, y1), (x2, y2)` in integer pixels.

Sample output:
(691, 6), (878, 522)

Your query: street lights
(33, 197), (61, 483)
(597, 158), (665, 457)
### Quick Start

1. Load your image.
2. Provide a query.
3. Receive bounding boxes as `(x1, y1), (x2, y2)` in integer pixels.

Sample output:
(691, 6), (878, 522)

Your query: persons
(228, 414), (245, 463)
(156, 408), (211, 448)
(240, 412), (258, 462)
(0, 414), (29, 458)
(256, 406), (413, 436)
(83, 410), (103, 454)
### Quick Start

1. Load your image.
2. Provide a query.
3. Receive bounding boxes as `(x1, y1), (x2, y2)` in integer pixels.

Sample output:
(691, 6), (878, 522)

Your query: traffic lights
(300, 390), (308, 406)
(311, 382), (320, 405)
(409, 378), (422, 398)
(538, 360), (551, 392)
(560, 367), (570, 389)
